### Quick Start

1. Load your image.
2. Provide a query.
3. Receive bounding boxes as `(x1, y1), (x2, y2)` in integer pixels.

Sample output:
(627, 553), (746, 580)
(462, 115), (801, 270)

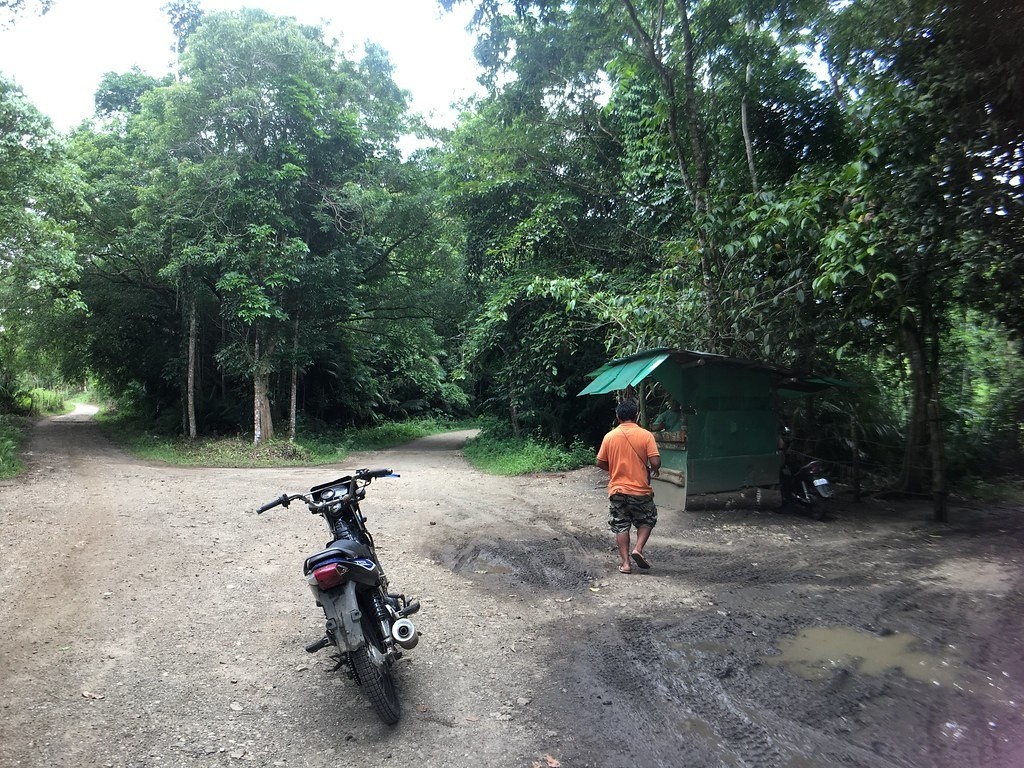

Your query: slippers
(618, 562), (631, 574)
(631, 552), (650, 571)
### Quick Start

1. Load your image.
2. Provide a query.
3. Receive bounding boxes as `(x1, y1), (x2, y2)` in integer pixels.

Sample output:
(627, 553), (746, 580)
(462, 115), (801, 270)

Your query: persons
(597, 401), (661, 574)
(652, 398), (682, 432)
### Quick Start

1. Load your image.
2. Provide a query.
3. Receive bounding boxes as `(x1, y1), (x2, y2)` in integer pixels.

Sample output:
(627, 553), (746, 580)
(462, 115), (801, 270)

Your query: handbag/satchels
(646, 465), (651, 486)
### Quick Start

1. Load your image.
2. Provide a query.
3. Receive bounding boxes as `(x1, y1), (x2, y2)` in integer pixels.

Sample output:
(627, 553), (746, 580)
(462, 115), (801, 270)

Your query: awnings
(577, 354), (669, 396)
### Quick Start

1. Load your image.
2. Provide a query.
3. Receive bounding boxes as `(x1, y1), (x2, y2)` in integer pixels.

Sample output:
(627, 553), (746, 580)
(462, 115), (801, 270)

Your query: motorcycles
(777, 426), (836, 521)
(256, 468), (422, 726)
